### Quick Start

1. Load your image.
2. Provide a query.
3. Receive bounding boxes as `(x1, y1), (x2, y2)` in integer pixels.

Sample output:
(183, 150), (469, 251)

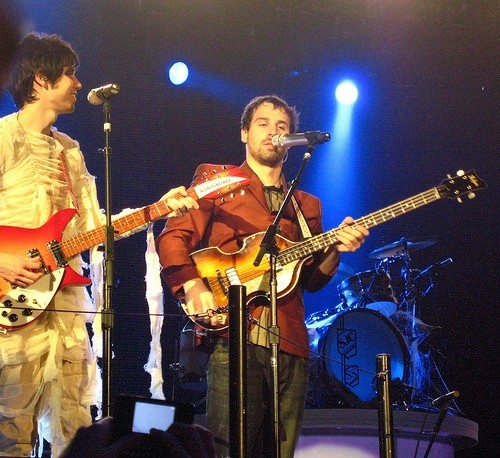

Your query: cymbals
(369, 235), (437, 259)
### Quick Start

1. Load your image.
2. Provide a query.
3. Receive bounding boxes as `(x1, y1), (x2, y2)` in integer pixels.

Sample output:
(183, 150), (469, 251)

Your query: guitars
(180, 168), (486, 330)
(0, 164), (251, 332)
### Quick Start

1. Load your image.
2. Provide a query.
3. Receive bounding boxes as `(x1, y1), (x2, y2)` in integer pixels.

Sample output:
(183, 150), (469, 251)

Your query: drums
(335, 269), (400, 320)
(300, 306), (411, 406)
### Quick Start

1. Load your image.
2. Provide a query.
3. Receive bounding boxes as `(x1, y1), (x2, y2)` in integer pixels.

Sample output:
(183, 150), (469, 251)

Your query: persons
(0, 31), (200, 458)
(154, 94), (369, 458)
(58, 416), (216, 458)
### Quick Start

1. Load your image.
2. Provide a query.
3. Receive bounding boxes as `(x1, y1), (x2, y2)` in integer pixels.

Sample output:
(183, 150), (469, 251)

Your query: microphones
(87, 83), (120, 105)
(416, 257), (453, 279)
(272, 132), (330, 149)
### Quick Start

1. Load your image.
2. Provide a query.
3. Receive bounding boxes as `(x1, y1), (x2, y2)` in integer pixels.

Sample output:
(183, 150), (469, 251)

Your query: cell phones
(112, 394), (195, 435)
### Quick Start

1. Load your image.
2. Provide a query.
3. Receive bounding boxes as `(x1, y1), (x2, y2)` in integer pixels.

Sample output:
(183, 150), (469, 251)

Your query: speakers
(305, 358), (338, 410)
